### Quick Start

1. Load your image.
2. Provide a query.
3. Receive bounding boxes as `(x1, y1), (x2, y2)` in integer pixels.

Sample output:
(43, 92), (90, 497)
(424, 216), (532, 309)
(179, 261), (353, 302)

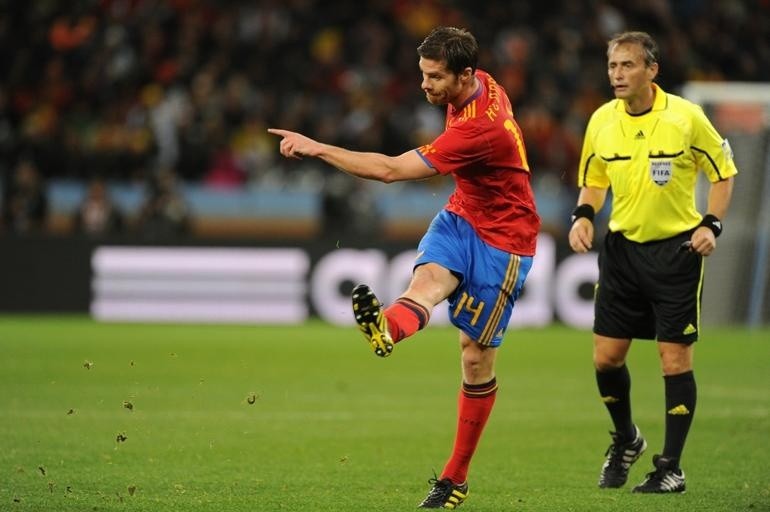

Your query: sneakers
(598, 424), (647, 488)
(352, 284), (394, 357)
(417, 469), (470, 508)
(632, 454), (685, 493)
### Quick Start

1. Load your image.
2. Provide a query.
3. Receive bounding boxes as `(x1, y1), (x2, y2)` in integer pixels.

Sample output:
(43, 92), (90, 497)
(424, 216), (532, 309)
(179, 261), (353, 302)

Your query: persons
(567, 32), (740, 495)
(266, 27), (544, 512)
(0, 1), (770, 315)
